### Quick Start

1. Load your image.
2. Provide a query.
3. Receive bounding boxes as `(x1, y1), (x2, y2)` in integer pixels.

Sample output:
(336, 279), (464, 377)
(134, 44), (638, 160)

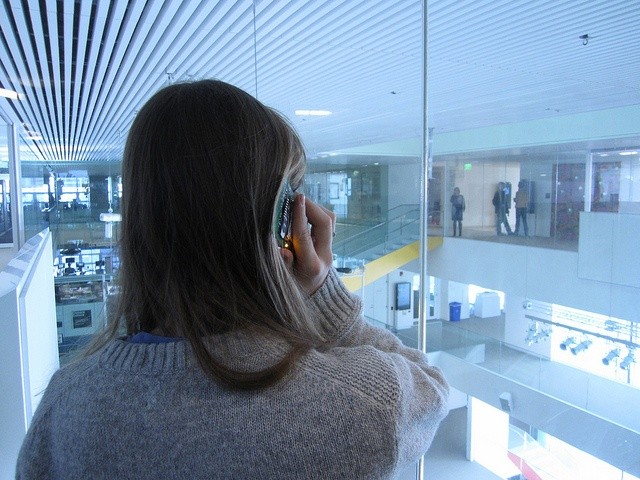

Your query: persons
(492, 182), (513, 236)
(513, 183), (529, 236)
(449, 186), (465, 237)
(16, 78), (453, 480)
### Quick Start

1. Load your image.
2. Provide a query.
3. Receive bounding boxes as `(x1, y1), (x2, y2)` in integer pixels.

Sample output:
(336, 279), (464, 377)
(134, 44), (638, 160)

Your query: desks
(475, 291), (501, 319)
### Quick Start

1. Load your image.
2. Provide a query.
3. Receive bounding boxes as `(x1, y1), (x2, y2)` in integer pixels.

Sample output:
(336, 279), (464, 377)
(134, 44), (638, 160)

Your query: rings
(332, 233), (336, 237)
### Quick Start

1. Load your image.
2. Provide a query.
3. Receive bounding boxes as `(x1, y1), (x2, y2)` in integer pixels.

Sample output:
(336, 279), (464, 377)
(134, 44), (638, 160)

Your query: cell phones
(271, 181), (310, 255)
